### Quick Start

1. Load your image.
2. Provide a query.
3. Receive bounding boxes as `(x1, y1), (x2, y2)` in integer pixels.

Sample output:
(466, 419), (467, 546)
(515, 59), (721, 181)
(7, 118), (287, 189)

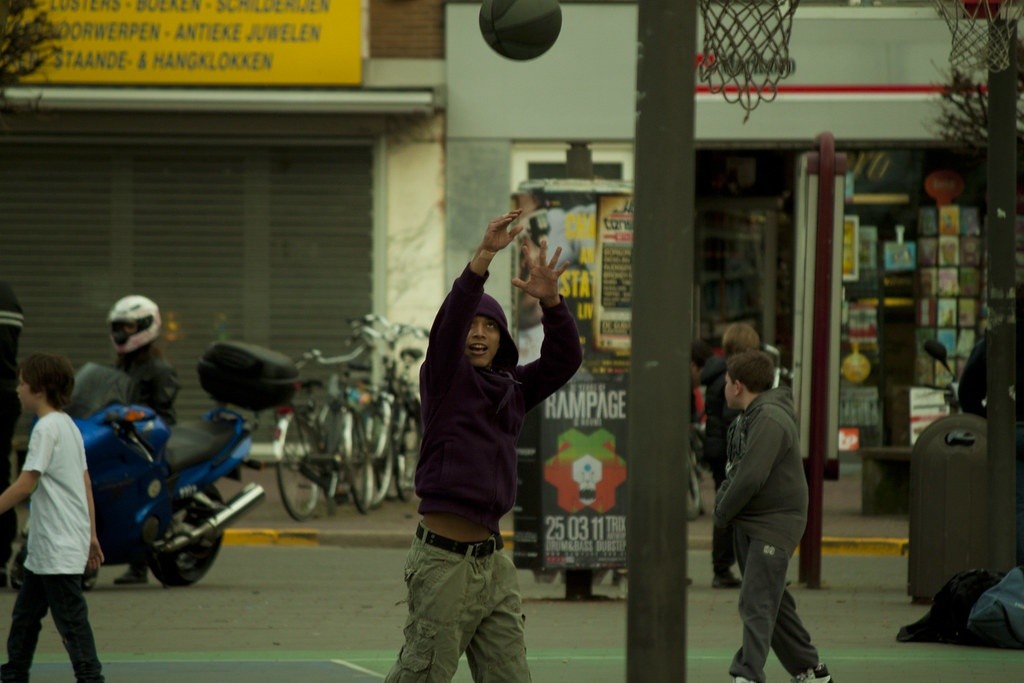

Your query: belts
(415, 522), (504, 559)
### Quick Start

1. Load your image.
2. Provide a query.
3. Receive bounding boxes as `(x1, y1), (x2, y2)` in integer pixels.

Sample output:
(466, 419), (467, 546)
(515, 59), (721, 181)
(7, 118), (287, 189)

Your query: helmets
(106, 294), (162, 354)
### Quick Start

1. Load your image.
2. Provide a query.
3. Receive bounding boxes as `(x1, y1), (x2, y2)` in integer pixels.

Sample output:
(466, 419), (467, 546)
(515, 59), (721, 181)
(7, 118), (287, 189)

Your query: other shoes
(733, 676), (756, 683)
(789, 662), (833, 683)
(112, 567), (147, 585)
(712, 574), (744, 589)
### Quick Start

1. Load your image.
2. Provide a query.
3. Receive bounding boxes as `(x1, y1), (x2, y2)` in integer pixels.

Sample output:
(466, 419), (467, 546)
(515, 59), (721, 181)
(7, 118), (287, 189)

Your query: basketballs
(479, 0), (562, 61)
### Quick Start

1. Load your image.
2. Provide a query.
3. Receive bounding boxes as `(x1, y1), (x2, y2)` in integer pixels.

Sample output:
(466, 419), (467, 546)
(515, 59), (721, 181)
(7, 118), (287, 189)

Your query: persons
(955, 320), (1015, 415)
(712, 348), (833, 683)
(689, 342), (712, 383)
(0, 354), (105, 683)
(382, 208), (583, 683)
(701, 324), (759, 589)
(106, 295), (179, 584)
(0, 282), (25, 586)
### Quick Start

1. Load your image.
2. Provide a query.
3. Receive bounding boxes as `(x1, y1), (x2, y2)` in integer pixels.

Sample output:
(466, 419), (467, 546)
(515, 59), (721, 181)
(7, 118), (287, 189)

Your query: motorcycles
(7, 337), (301, 594)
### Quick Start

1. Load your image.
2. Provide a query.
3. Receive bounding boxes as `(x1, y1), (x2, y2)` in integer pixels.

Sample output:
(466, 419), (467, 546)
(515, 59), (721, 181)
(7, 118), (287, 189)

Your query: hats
(475, 293), (519, 369)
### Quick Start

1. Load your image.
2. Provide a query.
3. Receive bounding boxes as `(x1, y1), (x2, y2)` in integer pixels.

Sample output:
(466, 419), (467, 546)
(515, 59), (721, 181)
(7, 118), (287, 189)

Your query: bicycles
(685, 407), (714, 520)
(271, 313), (431, 522)
